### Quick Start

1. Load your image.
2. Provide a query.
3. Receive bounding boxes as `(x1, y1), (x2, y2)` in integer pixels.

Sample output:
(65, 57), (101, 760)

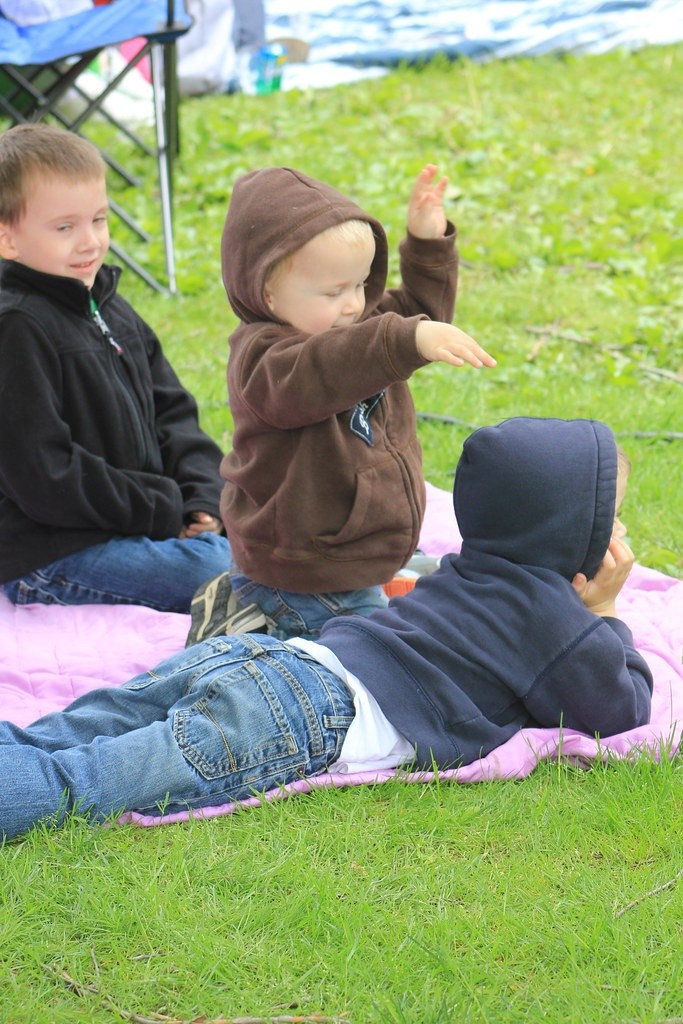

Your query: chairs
(0, 1), (202, 289)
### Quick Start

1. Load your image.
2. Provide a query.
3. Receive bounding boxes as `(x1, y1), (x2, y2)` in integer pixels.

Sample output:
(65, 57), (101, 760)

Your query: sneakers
(185, 572), (268, 649)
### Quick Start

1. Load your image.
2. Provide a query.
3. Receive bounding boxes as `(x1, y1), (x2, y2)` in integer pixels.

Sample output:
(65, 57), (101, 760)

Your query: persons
(1, 121), (236, 614)
(183, 165), (496, 648)
(1, 416), (654, 835)
